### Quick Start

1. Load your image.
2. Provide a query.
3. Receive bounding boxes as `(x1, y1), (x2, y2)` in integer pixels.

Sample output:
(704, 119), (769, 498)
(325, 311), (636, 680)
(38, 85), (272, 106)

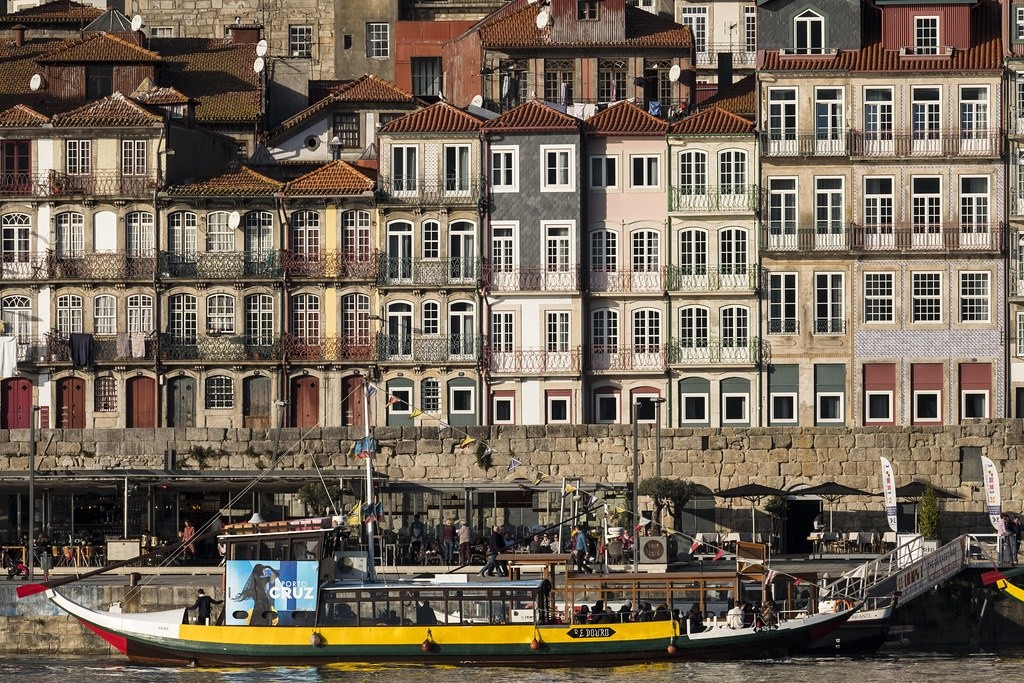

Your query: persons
(619, 530), (666, 564)
(727, 600), (777, 628)
(398, 514), (514, 577)
(998, 512), (1020, 565)
(389, 610), (400, 625)
(418, 601), (437, 624)
(178, 520), (195, 561)
(187, 588), (224, 626)
(530, 533), (558, 553)
(575, 524), (596, 574)
(548, 600), (703, 635)
(19, 532), (94, 566)
(814, 513), (825, 553)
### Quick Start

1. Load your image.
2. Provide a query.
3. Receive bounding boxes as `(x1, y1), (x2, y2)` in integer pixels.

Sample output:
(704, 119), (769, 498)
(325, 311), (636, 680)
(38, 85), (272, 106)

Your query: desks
(809, 532), (839, 553)
(726, 532), (762, 543)
(695, 533), (718, 555)
(842, 532), (876, 552)
(883, 531), (900, 554)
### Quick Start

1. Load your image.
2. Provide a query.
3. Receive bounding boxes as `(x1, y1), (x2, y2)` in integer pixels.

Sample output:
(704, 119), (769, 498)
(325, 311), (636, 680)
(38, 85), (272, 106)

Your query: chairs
(50, 546), (180, 568)
(691, 529), (909, 555)
(393, 533), (629, 566)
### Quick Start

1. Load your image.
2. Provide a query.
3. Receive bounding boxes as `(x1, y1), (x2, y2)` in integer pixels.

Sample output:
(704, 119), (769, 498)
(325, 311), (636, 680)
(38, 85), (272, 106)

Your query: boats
(15, 374), (901, 666)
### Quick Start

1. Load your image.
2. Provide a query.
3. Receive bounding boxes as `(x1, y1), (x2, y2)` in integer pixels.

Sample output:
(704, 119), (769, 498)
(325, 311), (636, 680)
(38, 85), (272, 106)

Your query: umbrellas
(868, 481), (965, 535)
(780, 482), (873, 533)
(715, 483), (786, 543)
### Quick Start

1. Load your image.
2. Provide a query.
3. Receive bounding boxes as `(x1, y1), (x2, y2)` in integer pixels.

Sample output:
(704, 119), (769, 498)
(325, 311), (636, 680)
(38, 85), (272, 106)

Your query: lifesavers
(833, 599), (850, 613)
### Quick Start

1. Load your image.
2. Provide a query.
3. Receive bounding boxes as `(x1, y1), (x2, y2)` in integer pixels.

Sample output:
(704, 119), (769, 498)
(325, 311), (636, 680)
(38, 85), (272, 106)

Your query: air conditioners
(334, 550), (368, 579)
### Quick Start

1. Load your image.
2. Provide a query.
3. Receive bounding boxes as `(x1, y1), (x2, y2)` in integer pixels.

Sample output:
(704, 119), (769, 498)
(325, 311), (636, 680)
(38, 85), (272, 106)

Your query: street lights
(649, 395), (667, 477)
(28, 405), (42, 582)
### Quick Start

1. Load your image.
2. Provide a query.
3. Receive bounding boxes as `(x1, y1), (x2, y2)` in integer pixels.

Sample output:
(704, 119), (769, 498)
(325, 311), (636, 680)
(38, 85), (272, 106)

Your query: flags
(347, 500), (385, 525)
(349, 436), (376, 459)
